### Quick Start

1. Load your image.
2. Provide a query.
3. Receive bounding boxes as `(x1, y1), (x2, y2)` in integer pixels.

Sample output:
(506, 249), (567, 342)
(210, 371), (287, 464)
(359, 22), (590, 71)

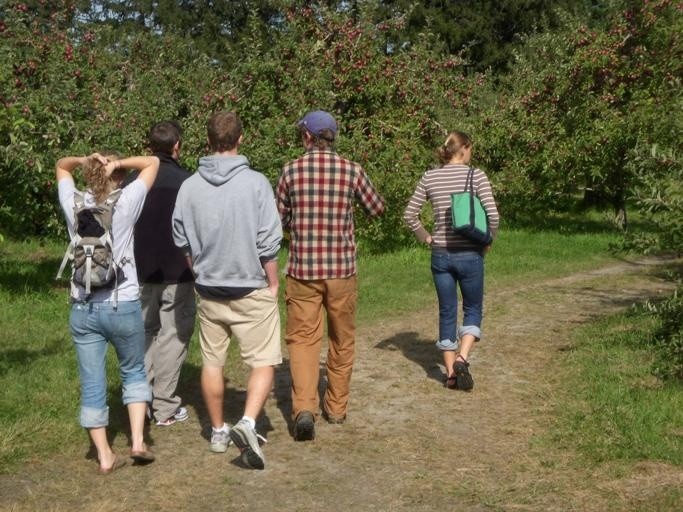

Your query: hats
(296, 110), (339, 148)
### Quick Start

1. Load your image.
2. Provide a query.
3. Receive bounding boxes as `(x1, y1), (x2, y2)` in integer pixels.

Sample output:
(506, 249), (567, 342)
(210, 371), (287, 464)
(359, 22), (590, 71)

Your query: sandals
(452, 354), (474, 391)
(444, 375), (457, 389)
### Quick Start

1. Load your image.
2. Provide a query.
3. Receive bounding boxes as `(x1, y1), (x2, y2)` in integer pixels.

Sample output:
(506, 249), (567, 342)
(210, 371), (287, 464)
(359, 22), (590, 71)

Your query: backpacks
(53, 190), (124, 304)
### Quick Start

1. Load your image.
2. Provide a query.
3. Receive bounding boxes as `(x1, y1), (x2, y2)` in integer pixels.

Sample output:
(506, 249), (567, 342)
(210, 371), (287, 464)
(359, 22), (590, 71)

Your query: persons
(54, 151), (160, 477)
(273, 110), (386, 441)
(169, 112), (285, 470)
(401, 130), (500, 391)
(118, 122), (197, 426)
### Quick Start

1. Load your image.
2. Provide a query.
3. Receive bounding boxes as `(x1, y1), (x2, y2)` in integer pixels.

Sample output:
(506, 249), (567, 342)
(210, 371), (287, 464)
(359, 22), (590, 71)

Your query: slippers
(98, 454), (127, 475)
(129, 442), (155, 462)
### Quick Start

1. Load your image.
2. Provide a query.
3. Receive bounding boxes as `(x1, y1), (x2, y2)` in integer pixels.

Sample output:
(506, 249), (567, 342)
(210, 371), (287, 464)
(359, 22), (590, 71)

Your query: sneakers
(155, 406), (189, 426)
(228, 419), (265, 469)
(208, 421), (235, 453)
(293, 410), (316, 441)
(323, 409), (347, 424)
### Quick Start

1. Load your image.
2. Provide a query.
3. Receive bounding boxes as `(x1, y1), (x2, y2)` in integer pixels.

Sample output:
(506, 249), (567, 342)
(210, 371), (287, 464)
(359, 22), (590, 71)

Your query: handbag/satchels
(449, 167), (494, 246)
